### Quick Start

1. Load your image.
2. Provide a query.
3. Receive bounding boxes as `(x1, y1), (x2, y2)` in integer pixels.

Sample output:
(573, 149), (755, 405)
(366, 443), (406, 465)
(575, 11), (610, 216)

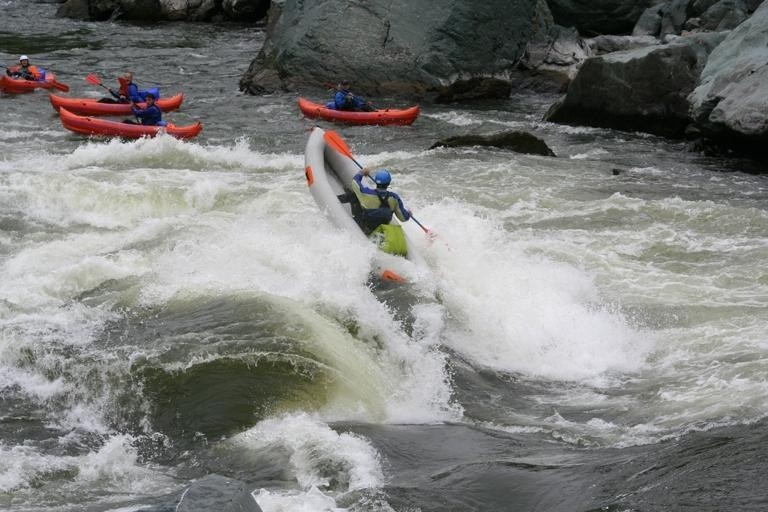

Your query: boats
(299, 97), (420, 126)
(2, 69), (56, 95)
(48, 92), (200, 140)
(305, 128), (432, 289)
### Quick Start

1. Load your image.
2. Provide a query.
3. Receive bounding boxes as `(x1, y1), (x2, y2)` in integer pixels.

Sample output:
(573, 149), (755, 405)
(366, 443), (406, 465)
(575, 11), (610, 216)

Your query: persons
(121, 93), (161, 126)
(337, 167), (412, 234)
(334, 79), (390, 112)
(6, 54), (40, 81)
(96, 72), (139, 105)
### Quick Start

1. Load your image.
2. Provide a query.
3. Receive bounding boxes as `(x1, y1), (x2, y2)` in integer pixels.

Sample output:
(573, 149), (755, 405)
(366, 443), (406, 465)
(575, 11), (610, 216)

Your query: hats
(341, 79), (350, 86)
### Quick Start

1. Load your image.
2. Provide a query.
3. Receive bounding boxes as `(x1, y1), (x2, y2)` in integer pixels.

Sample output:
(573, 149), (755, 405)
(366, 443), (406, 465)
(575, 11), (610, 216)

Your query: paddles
(86, 74), (144, 109)
(0, 64), (69, 92)
(324, 130), (438, 242)
(323, 82), (388, 109)
(118, 78), (141, 125)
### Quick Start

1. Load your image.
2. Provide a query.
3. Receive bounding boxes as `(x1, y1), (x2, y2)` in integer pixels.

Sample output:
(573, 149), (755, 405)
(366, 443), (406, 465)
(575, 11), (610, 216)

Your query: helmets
(374, 169), (392, 185)
(118, 72), (132, 84)
(19, 55), (30, 65)
